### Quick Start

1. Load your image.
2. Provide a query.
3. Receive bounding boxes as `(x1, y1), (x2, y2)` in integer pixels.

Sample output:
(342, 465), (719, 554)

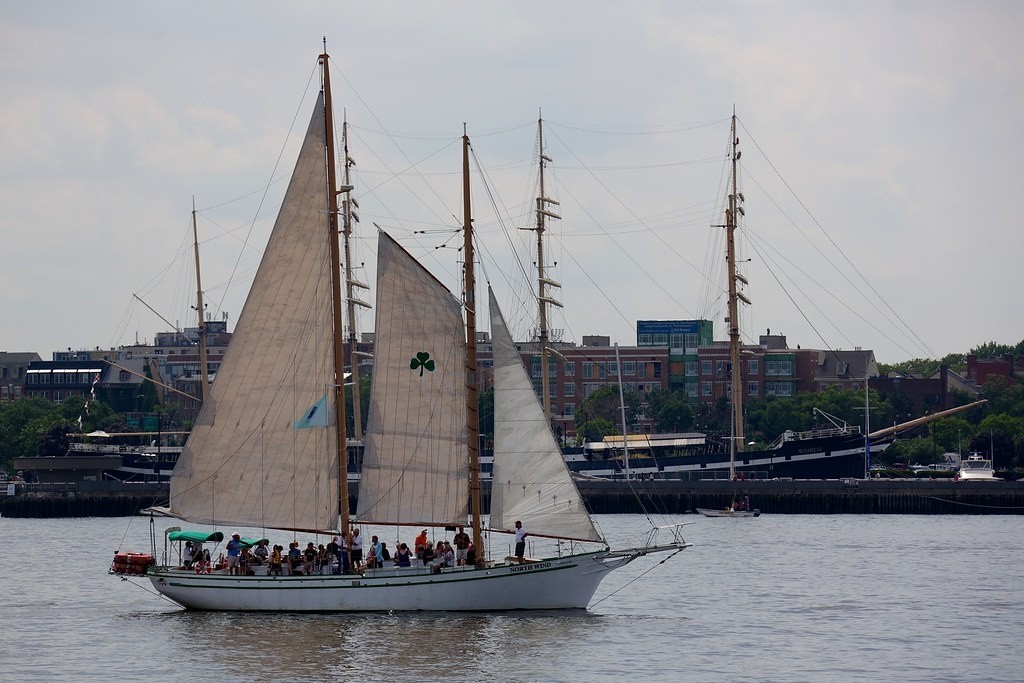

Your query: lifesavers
(196, 561), (211, 575)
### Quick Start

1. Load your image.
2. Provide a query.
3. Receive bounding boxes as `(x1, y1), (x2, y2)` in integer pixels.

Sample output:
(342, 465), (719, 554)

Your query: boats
(958, 425), (1000, 482)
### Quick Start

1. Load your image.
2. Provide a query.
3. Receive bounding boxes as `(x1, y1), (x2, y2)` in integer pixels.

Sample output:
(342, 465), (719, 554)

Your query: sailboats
(107, 32), (697, 614)
(64, 102), (991, 480)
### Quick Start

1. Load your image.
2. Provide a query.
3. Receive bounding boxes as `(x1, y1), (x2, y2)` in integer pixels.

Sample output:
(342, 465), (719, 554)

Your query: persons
(649, 472), (654, 481)
(632, 471), (636, 481)
(507, 521), (528, 563)
(394, 543), (413, 566)
(751, 471), (755, 481)
(281, 529), (390, 576)
(954, 473), (960, 481)
(415, 529), (455, 574)
(734, 472), (738, 481)
(640, 473), (645, 481)
(454, 526), (485, 569)
(741, 472), (744, 482)
(877, 471), (880, 478)
(226, 534), (269, 575)
(268, 545), (283, 575)
(183, 542), (194, 569)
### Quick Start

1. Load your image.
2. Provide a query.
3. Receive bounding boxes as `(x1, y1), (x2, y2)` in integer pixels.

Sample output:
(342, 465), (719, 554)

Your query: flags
(78, 376), (100, 428)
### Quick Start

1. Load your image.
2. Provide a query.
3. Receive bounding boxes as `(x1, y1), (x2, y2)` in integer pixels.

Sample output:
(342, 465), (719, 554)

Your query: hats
(232, 532), (237, 536)
(514, 521), (521, 524)
(422, 528), (428, 532)
(185, 541), (193, 545)
